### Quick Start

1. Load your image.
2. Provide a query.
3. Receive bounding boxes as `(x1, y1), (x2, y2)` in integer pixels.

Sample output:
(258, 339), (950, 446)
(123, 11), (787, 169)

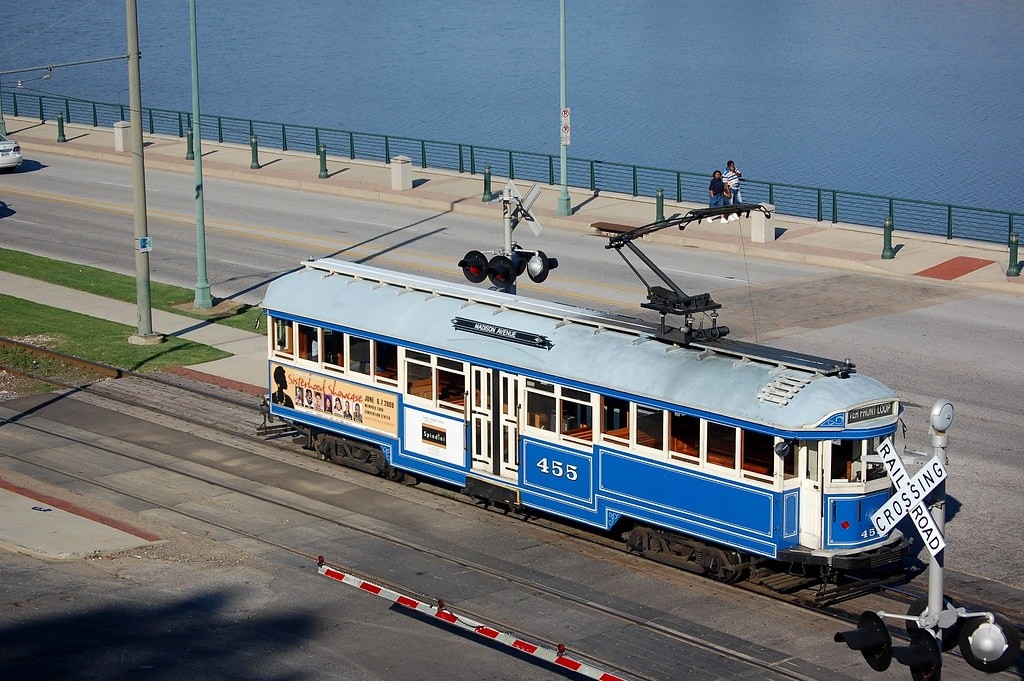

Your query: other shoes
(728, 215), (735, 221)
(706, 217), (712, 223)
(720, 218), (728, 223)
(733, 213), (740, 219)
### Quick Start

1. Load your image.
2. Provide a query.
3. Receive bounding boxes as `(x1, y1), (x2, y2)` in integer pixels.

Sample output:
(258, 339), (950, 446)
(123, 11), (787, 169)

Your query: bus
(257, 256), (908, 592)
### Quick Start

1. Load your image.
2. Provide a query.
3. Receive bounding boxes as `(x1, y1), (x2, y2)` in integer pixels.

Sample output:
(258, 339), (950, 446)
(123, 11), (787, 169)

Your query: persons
(295, 387), (304, 406)
(333, 397), (342, 418)
(304, 389), (313, 409)
(324, 396), (332, 414)
(353, 403), (362, 423)
(272, 366), (295, 409)
(314, 392), (323, 412)
(343, 401), (352, 420)
(707, 160), (742, 223)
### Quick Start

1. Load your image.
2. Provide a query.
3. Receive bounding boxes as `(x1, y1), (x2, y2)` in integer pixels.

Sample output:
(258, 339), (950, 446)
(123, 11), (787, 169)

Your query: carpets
(912, 256), (997, 282)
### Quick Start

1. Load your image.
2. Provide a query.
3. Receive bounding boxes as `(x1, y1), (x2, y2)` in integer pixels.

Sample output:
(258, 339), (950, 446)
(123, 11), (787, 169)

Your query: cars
(0, 133), (22, 170)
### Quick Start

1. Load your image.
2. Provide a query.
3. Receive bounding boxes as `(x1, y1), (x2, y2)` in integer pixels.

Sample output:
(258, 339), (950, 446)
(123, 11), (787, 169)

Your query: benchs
(407, 376), (480, 413)
(562, 411), (664, 448)
